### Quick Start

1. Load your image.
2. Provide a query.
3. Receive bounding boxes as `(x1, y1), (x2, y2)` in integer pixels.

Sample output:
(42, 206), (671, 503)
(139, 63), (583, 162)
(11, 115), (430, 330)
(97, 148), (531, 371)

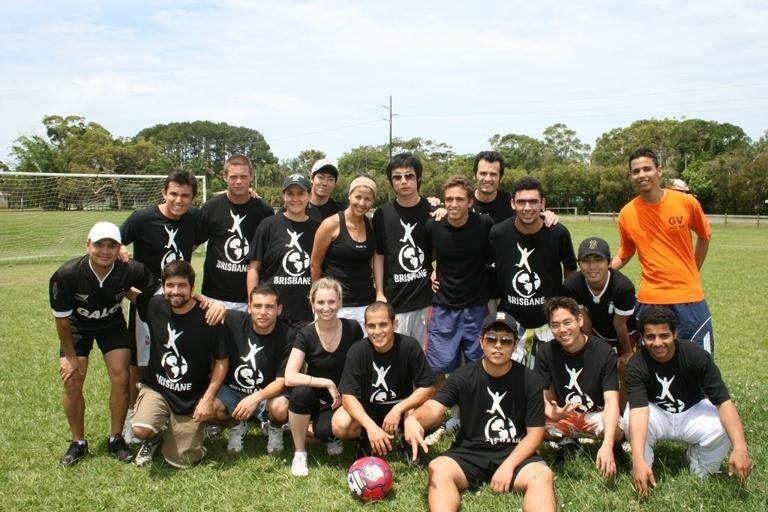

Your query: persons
(48, 222), (213, 467)
(405, 311), (558, 512)
(620, 306), (753, 494)
(117, 149), (576, 477)
(532, 296), (624, 480)
(609, 147), (714, 361)
(562, 238), (639, 415)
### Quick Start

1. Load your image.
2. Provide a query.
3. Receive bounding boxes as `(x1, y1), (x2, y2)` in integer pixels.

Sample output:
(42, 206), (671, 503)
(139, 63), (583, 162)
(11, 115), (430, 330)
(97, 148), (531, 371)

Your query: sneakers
(108, 411), (163, 463)
(550, 437), (581, 465)
(358, 430), (375, 460)
(613, 442), (632, 474)
(291, 452), (309, 478)
(399, 417), (460, 465)
(60, 440), (88, 468)
(327, 438), (344, 456)
(262, 419), (286, 456)
(683, 446), (710, 480)
(201, 419), (250, 459)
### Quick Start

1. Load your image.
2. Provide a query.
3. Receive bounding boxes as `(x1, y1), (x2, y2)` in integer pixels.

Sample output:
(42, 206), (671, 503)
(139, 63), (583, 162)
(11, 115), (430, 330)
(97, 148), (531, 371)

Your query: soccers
(348, 456), (393, 502)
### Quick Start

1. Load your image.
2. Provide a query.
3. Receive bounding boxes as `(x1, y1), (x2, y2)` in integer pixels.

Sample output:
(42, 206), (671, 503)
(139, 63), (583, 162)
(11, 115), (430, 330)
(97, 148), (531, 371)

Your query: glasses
(390, 173), (416, 184)
(485, 336), (513, 348)
(550, 317), (577, 330)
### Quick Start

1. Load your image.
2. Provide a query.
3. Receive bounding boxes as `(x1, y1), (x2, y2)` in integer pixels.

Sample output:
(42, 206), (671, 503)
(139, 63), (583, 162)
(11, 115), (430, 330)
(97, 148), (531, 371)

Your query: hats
(282, 173), (311, 191)
(87, 220), (122, 244)
(578, 237), (611, 259)
(311, 159), (340, 177)
(481, 311), (520, 337)
